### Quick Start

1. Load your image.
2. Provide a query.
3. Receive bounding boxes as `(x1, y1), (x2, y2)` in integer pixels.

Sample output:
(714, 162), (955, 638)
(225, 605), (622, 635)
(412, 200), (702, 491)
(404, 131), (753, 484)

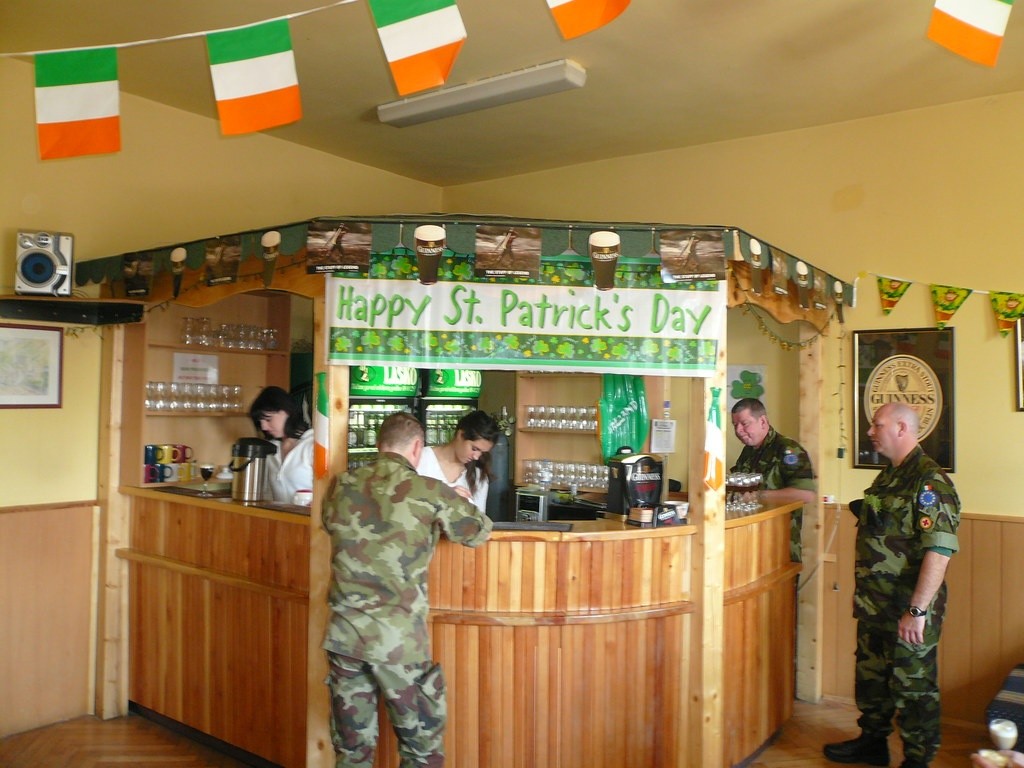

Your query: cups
(749, 238), (763, 294)
(835, 281), (844, 323)
(170, 248), (187, 299)
(796, 260), (810, 308)
(588, 230), (621, 291)
(414, 225), (447, 286)
(261, 230), (281, 288)
(144, 444), (198, 483)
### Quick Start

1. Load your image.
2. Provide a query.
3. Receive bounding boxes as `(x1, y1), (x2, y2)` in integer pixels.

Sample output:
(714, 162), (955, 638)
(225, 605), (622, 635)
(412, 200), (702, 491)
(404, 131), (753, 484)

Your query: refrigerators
(290, 350), (423, 472)
(421, 367), (481, 452)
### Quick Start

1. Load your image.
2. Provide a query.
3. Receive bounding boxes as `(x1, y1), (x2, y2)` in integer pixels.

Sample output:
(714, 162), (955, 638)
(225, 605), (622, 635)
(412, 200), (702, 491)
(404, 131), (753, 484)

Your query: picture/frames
(0, 322), (64, 409)
(853, 326), (956, 473)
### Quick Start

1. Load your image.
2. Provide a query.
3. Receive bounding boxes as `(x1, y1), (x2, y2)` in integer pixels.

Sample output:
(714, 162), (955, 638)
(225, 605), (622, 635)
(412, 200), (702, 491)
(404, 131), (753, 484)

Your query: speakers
(14, 232), (75, 297)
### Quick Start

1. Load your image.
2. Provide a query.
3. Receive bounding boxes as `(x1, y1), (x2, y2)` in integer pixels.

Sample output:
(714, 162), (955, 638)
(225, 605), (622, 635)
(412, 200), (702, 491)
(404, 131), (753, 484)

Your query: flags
(369, 0), (467, 96)
(205, 17), (303, 136)
(547, 0), (631, 40)
(34, 45), (122, 161)
(925, 0), (1015, 70)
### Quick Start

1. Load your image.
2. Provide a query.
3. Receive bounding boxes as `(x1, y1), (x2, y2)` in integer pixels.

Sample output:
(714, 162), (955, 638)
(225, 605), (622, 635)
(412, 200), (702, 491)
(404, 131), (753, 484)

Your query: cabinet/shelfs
(120, 291), (293, 487)
(513, 371), (671, 494)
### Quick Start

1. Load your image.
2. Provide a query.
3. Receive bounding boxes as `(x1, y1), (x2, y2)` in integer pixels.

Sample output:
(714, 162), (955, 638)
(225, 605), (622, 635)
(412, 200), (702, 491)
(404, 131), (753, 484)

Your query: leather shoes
(900, 758), (928, 768)
(824, 730), (889, 764)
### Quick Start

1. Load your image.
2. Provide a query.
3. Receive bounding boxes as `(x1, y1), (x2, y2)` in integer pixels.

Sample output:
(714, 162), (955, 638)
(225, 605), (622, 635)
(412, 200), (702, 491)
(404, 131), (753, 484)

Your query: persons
(730, 398), (817, 563)
(246, 383), (315, 507)
(415, 410), (501, 515)
(821, 403), (961, 768)
(321, 410), (492, 767)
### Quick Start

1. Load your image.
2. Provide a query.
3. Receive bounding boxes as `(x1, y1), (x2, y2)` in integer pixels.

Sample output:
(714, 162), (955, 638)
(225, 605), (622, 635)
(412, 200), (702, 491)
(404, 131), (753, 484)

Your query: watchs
(907, 604), (928, 617)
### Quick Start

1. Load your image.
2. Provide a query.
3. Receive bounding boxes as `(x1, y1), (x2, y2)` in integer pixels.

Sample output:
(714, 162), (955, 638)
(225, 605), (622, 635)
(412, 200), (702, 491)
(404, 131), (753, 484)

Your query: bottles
(425, 409), (468, 446)
(539, 472), (550, 491)
(347, 404), (418, 471)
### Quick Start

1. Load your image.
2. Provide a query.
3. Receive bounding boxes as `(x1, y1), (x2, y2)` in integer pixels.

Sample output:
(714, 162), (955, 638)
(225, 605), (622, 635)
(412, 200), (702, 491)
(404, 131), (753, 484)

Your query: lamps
(376, 59), (588, 128)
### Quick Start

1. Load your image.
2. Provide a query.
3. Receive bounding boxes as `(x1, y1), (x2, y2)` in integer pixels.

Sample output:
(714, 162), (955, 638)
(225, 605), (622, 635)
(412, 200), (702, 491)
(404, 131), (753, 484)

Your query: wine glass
(521, 405), (598, 430)
(144, 381), (243, 412)
(725, 472), (764, 511)
(181, 315), (278, 351)
(199, 465), (215, 496)
(522, 459), (618, 488)
